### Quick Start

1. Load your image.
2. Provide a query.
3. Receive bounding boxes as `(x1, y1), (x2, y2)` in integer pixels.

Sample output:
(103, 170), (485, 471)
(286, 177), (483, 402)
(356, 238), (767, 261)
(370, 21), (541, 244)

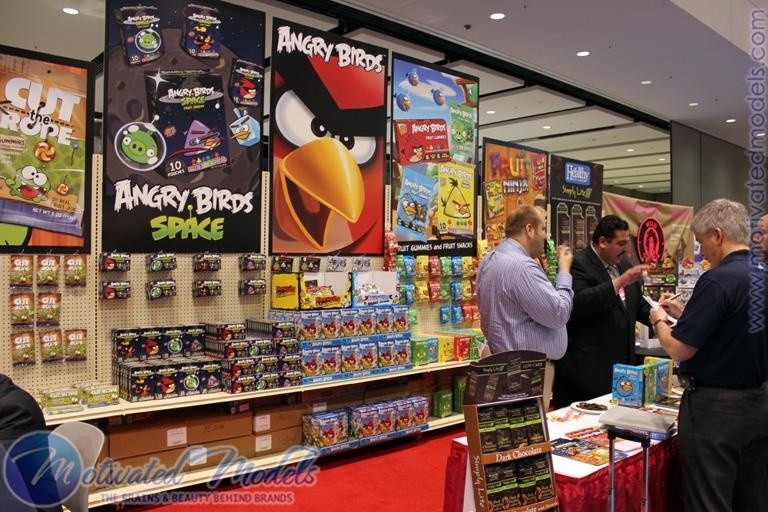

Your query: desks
(443, 376), (685, 512)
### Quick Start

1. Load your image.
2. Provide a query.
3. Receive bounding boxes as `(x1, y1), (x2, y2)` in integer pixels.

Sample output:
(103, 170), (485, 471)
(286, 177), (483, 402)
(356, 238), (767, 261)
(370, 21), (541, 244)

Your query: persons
(649, 198), (768, 512)
(570, 215), (654, 411)
(0, 373), (63, 512)
(476, 205), (574, 412)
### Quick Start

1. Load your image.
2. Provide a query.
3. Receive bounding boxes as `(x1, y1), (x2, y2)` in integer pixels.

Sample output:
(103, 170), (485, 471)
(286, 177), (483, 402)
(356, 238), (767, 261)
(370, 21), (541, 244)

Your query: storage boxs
(82, 398), (344, 492)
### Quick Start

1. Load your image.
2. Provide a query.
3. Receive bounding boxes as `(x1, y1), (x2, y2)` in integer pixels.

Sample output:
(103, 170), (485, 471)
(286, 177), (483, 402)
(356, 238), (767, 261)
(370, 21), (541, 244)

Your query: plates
(571, 401), (607, 414)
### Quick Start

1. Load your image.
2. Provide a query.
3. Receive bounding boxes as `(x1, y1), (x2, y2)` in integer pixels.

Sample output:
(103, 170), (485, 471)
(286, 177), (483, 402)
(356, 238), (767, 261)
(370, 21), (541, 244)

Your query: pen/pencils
(659, 292), (682, 306)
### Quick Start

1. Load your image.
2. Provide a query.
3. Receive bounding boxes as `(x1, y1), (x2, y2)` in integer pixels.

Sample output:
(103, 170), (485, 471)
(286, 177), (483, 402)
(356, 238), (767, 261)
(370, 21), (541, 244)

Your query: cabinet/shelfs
(41, 357), (477, 510)
